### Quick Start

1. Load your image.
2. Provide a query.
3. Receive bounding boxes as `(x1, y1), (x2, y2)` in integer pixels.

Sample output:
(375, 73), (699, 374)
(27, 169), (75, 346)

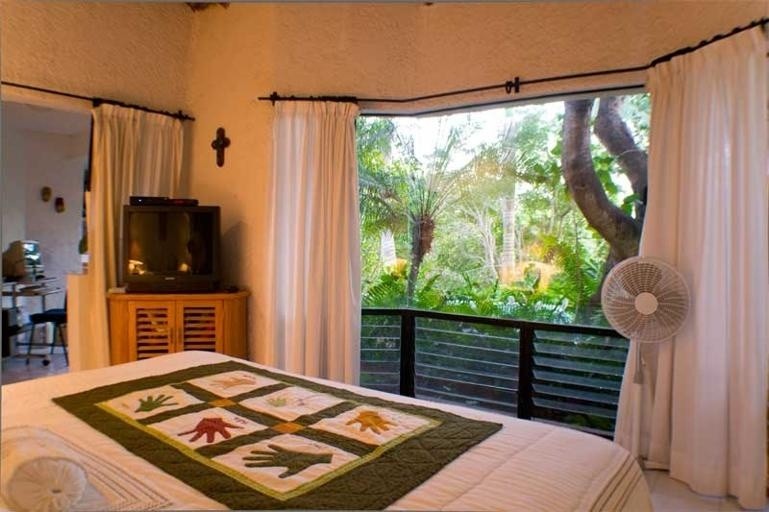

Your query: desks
(2, 277), (63, 365)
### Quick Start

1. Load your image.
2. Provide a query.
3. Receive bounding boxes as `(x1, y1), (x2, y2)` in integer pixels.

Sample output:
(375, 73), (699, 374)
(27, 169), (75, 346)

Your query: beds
(0, 350), (655, 512)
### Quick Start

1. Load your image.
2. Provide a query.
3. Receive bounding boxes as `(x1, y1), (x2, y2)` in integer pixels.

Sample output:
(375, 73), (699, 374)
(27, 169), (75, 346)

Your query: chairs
(25, 289), (68, 364)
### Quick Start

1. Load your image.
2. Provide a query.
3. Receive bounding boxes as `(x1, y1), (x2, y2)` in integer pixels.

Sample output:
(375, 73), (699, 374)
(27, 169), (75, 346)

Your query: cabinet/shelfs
(108, 298), (248, 367)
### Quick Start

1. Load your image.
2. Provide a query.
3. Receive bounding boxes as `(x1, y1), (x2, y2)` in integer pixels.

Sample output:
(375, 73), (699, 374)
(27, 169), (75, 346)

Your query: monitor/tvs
(122, 204), (222, 292)
(3, 240), (46, 282)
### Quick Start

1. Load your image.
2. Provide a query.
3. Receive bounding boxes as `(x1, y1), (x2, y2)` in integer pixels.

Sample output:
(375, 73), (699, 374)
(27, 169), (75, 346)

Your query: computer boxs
(2, 307), (17, 356)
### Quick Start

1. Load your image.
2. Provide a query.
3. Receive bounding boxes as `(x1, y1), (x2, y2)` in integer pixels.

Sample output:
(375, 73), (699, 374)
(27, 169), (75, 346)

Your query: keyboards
(19, 286), (49, 292)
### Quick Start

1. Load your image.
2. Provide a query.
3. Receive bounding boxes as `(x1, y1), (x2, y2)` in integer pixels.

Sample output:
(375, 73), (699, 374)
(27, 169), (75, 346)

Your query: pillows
(0, 412), (88, 512)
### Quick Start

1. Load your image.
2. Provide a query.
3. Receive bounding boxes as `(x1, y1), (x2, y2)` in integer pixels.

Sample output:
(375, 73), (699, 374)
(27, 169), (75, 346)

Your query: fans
(600, 255), (691, 464)
